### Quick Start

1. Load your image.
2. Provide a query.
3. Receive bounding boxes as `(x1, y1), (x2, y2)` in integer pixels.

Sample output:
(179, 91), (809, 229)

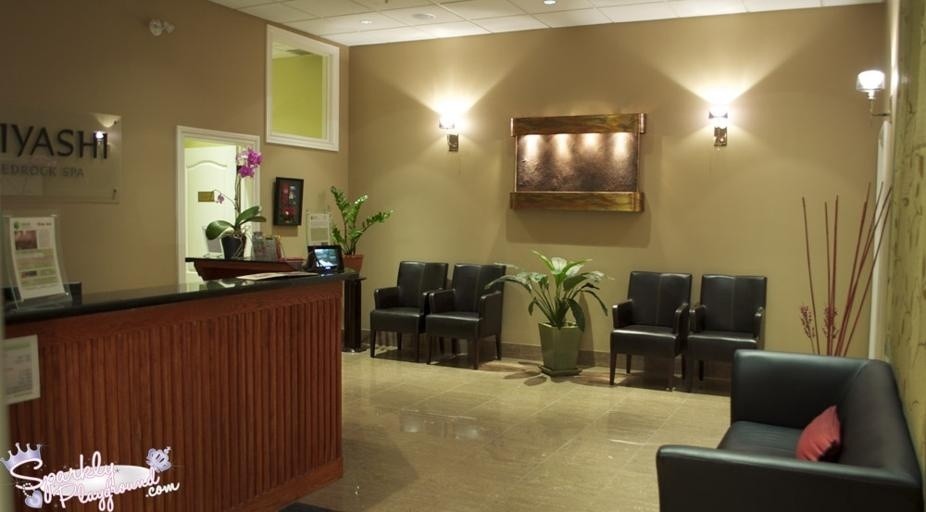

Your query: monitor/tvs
(303, 244), (344, 275)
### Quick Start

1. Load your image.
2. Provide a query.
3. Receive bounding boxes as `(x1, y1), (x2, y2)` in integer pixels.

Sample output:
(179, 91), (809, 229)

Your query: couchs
(656, 352), (925, 512)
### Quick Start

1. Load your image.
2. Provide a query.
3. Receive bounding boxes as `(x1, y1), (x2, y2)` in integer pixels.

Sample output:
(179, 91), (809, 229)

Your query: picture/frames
(507, 111), (645, 214)
(273, 177), (304, 226)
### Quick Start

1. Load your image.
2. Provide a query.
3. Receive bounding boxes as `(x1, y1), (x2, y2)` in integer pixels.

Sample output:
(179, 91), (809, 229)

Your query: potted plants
(485, 249), (613, 377)
(327, 185), (396, 275)
(252, 230), (283, 261)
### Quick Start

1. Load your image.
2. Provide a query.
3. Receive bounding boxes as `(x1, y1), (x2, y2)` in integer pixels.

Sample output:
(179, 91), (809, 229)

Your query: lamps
(438, 114), (460, 152)
(707, 106), (733, 142)
(853, 70), (890, 117)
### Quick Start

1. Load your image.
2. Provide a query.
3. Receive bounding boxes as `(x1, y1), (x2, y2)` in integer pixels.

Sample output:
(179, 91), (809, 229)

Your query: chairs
(425, 262), (506, 369)
(606, 270), (691, 387)
(688, 273), (767, 381)
(371, 259), (448, 363)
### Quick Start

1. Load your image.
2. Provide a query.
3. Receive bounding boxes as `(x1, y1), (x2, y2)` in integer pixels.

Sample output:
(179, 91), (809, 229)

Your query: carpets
(278, 502), (339, 512)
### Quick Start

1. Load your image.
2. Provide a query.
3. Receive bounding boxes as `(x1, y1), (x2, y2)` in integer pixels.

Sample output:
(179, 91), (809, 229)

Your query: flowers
(206, 144), (266, 239)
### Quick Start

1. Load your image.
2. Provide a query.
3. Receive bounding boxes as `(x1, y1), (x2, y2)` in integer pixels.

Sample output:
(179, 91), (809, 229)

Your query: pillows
(797, 405), (842, 461)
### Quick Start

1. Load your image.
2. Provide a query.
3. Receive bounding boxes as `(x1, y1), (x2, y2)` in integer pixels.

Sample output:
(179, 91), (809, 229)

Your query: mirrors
(264, 22), (343, 152)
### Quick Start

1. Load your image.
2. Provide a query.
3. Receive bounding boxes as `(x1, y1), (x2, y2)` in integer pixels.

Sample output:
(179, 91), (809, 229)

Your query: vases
(220, 235), (246, 260)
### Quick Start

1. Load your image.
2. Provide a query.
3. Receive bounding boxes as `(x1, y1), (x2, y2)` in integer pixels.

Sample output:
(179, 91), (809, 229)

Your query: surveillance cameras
(150, 18), (176, 36)
